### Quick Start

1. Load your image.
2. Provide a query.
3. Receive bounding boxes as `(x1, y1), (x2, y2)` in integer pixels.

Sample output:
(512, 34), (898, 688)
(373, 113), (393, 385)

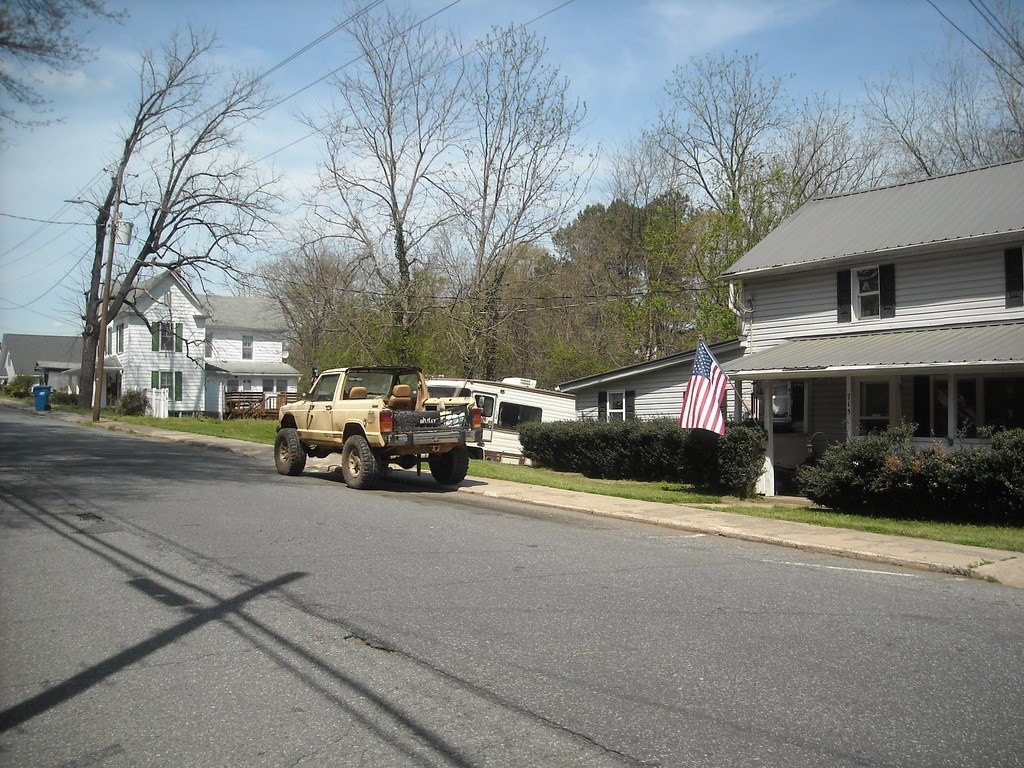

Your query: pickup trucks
(274, 367), (485, 490)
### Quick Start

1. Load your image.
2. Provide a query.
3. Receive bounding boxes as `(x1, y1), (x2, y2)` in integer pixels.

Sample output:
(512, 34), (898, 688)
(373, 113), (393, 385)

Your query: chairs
(349, 383), (412, 409)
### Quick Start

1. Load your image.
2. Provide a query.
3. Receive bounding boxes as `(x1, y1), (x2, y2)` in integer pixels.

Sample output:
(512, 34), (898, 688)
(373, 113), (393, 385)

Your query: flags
(679, 338), (730, 435)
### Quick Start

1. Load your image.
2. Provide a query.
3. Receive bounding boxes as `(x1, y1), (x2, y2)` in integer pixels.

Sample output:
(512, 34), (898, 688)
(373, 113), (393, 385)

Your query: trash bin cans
(33, 386), (52, 411)
